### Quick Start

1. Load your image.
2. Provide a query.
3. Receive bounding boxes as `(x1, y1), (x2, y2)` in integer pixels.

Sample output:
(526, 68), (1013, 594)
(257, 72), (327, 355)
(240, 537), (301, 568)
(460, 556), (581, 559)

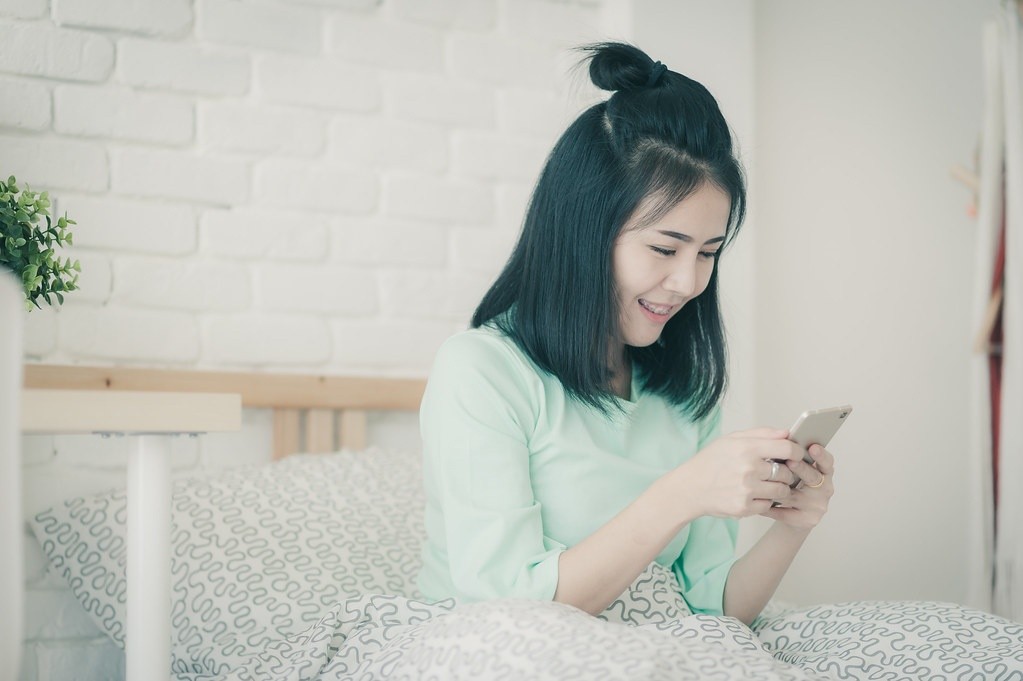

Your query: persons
(419, 43), (835, 624)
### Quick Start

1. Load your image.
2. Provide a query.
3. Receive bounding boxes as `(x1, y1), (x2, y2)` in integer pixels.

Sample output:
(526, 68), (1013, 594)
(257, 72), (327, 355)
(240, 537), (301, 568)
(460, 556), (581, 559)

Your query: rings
(766, 459), (778, 480)
(811, 475), (825, 487)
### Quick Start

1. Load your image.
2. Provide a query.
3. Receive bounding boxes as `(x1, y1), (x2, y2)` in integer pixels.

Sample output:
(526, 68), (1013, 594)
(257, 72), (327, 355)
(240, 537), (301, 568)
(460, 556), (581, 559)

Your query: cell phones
(771, 404), (853, 507)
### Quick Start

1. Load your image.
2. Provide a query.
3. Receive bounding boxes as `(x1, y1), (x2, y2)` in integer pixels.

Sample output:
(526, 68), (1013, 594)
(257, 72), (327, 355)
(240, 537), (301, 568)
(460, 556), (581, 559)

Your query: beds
(16, 363), (1023, 681)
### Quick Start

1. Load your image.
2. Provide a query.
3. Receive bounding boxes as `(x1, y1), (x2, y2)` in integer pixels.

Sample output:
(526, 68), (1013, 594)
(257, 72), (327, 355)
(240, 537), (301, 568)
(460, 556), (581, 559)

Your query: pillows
(21, 441), (433, 674)
